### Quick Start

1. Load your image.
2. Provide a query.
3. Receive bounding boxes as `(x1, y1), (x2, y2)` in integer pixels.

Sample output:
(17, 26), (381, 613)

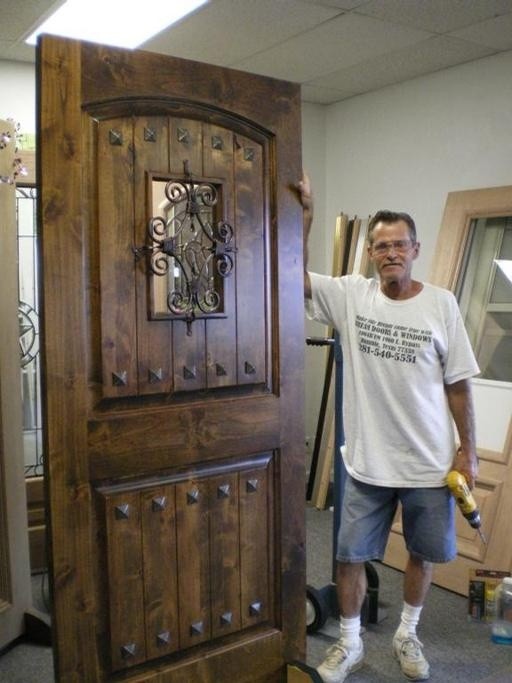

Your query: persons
(299, 174), (480, 681)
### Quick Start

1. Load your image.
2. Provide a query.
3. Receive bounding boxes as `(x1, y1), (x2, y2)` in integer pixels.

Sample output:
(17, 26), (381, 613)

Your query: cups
(373, 240), (412, 251)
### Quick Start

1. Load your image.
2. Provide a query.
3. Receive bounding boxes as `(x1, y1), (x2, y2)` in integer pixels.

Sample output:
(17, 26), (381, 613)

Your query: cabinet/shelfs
(491, 577), (512, 645)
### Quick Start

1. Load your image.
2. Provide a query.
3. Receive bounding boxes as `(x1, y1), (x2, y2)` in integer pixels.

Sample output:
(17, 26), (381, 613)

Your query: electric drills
(445, 446), (488, 544)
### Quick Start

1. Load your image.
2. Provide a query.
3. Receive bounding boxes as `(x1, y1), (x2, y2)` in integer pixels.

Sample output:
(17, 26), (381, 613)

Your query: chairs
(392, 633), (430, 680)
(316, 637), (363, 683)
